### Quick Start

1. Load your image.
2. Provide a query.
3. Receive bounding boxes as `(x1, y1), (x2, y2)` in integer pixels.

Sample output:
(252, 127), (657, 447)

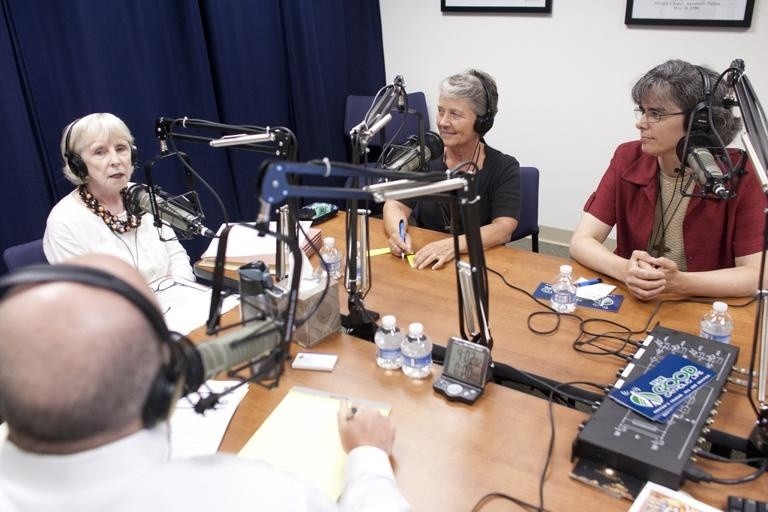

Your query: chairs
(343, 91), (429, 210)
(511, 167), (538, 256)
(3, 237), (50, 272)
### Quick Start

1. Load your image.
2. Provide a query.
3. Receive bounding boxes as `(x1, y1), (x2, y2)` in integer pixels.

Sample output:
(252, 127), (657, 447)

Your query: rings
(392, 245), (397, 250)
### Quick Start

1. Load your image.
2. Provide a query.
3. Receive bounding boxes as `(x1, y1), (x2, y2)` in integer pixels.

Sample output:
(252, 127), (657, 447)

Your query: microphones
(678, 134), (730, 200)
(167, 324), (282, 397)
(124, 184), (215, 239)
(389, 131), (444, 171)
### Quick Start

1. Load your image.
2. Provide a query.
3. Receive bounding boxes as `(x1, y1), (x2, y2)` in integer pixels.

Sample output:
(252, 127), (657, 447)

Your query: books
(198, 221), (324, 291)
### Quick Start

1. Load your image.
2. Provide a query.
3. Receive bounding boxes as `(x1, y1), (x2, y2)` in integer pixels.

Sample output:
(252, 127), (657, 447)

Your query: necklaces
(77, 184), (143, 233)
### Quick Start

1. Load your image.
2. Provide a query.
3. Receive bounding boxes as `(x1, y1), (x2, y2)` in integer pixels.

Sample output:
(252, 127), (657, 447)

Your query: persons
(1, 253), (410, 512)
(568, 58), (767, 303)
(383, 69), (523, 270)
(42, 112), (196, 293)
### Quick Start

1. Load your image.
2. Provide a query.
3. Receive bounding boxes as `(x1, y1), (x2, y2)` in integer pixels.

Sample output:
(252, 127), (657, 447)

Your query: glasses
(635, 107), (686, 122)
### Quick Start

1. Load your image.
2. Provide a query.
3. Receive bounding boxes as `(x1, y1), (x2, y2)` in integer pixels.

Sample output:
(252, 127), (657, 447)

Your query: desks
(177, 303), (768, 512)
(194, 210), (768, 460)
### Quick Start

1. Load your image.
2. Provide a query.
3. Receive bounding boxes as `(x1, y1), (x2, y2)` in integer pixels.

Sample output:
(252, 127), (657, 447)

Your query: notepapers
(407, 254), (417, 268)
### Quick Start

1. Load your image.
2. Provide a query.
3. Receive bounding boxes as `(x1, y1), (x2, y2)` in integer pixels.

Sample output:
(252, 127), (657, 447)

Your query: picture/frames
(441, 0), (552, 14)
(625, 0), (754, 28)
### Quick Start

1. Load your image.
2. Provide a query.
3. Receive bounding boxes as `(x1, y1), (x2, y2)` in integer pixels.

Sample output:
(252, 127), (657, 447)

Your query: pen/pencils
(572, 277), (602, 288)
(347, 406), (357, 420)
(399, 219), (404, 260)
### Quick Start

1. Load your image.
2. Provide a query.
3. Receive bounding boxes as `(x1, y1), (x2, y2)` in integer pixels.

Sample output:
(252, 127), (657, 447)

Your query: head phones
(65, 120), (138, 182)
(468, 70), (493, 135)
(677, 66), (719, 168)
(0, 265), (187, 427)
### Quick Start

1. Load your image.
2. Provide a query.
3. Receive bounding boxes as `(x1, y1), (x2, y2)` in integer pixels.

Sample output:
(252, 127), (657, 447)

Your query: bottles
(402, 324), (432, 379)
(376, 315), (402, 369)
(551, 265), (577, 314)
(319, 237), (341, 280)
(700, 301), (733, 343)
